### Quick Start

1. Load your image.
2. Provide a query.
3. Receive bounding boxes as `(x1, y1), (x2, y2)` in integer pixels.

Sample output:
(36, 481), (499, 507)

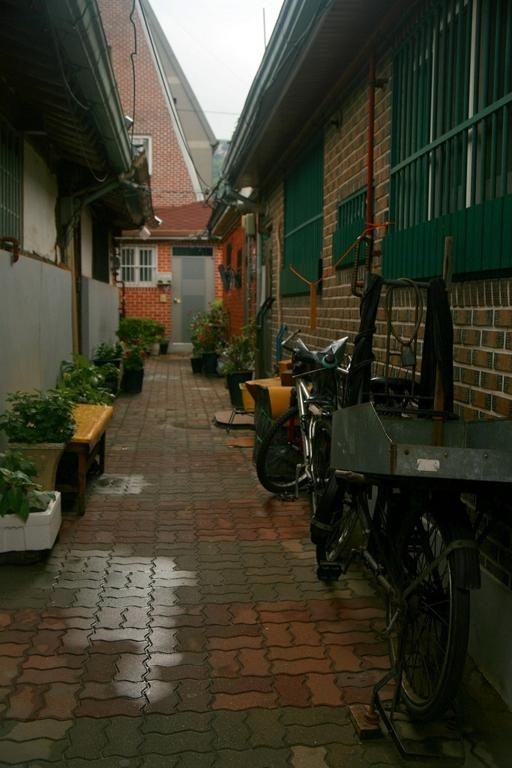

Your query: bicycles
(254, 274), (512, 768)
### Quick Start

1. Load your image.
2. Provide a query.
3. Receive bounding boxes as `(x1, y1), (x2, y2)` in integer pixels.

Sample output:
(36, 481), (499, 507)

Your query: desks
(55, 403), (114, 516)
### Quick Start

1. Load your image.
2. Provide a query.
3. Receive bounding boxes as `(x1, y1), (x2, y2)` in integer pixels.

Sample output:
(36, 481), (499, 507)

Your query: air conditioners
(156, 272), (173, 285)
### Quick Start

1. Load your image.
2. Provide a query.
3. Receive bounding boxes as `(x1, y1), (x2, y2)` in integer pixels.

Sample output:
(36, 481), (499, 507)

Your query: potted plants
(0, 447), (62, 554)
(0, 385), (79, 492)
(92, 316), (255, 407)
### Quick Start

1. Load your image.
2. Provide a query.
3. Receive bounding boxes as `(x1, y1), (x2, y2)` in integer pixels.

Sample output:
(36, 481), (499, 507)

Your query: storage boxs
(239, 376), (281, 413)
